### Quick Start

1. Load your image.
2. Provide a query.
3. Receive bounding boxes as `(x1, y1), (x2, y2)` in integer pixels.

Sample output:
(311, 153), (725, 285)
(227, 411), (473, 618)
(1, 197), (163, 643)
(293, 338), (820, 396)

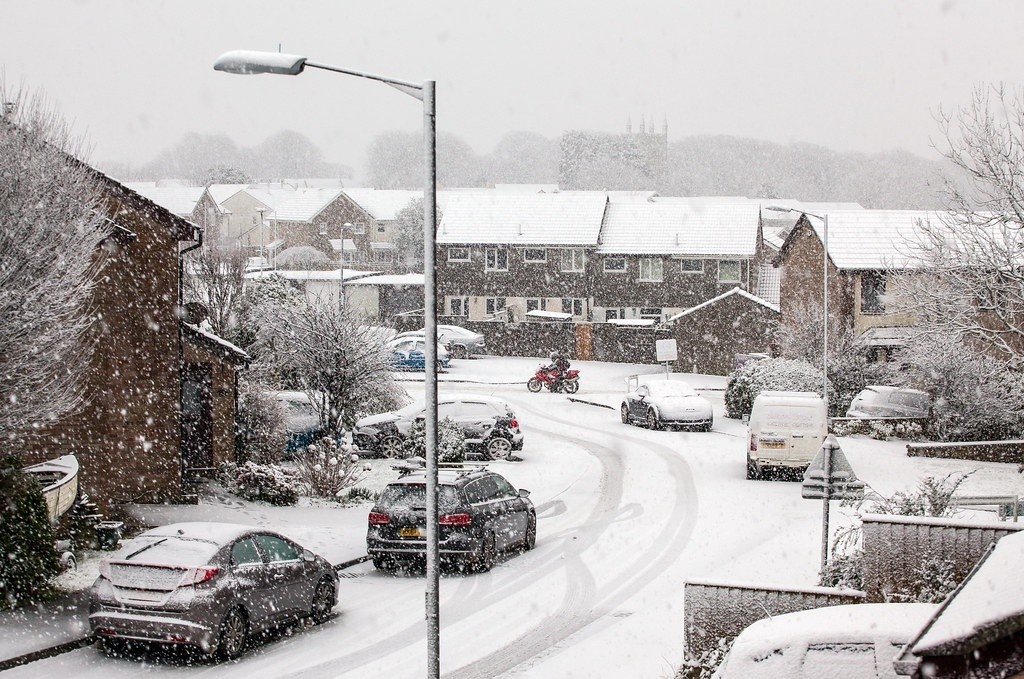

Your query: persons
(547, 352), (565, 386)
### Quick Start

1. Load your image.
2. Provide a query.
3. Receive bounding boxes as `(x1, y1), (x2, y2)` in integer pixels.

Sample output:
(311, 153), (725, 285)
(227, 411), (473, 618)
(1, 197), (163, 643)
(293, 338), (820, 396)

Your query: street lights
(212, 49), (439, 679)
(338, 223), (353, 317)
(255, 207), (269, 279)
(766, 205), (829, 400)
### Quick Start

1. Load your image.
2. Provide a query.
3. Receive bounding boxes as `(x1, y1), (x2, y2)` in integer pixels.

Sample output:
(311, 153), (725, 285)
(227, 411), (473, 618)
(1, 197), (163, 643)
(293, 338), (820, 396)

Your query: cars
(243, 256), (272, 274)
(388, 330), (455, 363)
(621, 381), (714, 432)
(88, 521), (341, 663)
(844, 384), (953, 420)
(379, 337), (453, 374)
(417, 324), (487, 360)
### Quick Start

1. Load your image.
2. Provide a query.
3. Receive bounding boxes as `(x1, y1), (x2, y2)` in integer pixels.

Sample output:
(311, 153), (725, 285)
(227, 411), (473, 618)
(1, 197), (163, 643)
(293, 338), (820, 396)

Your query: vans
(741, 390), (828, 483)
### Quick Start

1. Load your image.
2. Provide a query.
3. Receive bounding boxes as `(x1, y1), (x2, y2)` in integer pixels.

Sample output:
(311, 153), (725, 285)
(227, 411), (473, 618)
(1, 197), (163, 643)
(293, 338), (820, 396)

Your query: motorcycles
(527, 360), (582, 395)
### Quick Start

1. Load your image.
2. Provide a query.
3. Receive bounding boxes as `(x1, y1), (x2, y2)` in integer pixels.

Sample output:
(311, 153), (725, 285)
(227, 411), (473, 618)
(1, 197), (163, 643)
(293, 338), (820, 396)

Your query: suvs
(235, 390), (342, 463)
(351, 394), (523, 462)
(367, 463), (537, 575)
